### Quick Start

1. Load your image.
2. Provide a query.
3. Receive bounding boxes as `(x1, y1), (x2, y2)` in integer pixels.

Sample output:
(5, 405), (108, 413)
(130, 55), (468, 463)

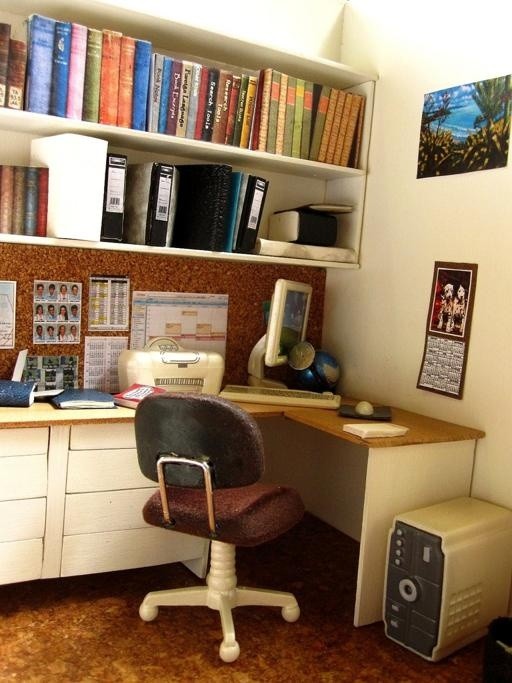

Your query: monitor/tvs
(247, 278), (313, 391)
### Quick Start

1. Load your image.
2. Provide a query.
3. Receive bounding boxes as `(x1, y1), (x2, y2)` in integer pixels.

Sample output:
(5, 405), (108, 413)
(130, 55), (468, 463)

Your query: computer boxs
(382, 495), (512, 662)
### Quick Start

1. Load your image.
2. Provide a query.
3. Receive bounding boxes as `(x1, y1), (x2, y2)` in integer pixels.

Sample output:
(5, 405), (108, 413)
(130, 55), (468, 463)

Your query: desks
(0, 385), (485, 627)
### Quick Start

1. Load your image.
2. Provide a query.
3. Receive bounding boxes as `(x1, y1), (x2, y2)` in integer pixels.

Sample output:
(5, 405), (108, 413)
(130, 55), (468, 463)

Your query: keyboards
(219, 384), (342, 410)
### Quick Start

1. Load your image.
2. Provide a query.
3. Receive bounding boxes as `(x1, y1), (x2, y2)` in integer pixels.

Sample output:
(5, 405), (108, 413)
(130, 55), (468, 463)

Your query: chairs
(133, 389), (309, 663)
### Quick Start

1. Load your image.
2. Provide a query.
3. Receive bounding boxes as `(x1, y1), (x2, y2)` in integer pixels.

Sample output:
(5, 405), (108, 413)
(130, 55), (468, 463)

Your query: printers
(117, 337), (225, 396)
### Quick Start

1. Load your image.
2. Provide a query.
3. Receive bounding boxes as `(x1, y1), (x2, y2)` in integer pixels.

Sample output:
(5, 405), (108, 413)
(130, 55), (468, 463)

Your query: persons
(34, 282), (81, 342)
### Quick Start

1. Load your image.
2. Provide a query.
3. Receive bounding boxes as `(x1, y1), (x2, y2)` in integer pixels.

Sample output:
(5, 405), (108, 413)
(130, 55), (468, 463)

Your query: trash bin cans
(482, 616), (512, 683)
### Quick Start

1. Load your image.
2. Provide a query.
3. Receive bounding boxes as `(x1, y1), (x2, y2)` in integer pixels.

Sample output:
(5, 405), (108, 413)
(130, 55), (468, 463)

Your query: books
(341, 420), (408, 437)
(113, 381), (170, 411)
(52, 386), (117, 409)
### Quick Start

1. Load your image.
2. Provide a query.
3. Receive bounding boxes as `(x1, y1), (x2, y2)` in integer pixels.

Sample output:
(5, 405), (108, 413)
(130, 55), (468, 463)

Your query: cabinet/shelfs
(1, 0), (382, 271)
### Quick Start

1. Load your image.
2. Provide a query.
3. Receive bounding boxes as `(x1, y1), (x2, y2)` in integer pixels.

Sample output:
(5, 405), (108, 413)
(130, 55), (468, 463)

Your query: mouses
(355, 401), (374, 416)
